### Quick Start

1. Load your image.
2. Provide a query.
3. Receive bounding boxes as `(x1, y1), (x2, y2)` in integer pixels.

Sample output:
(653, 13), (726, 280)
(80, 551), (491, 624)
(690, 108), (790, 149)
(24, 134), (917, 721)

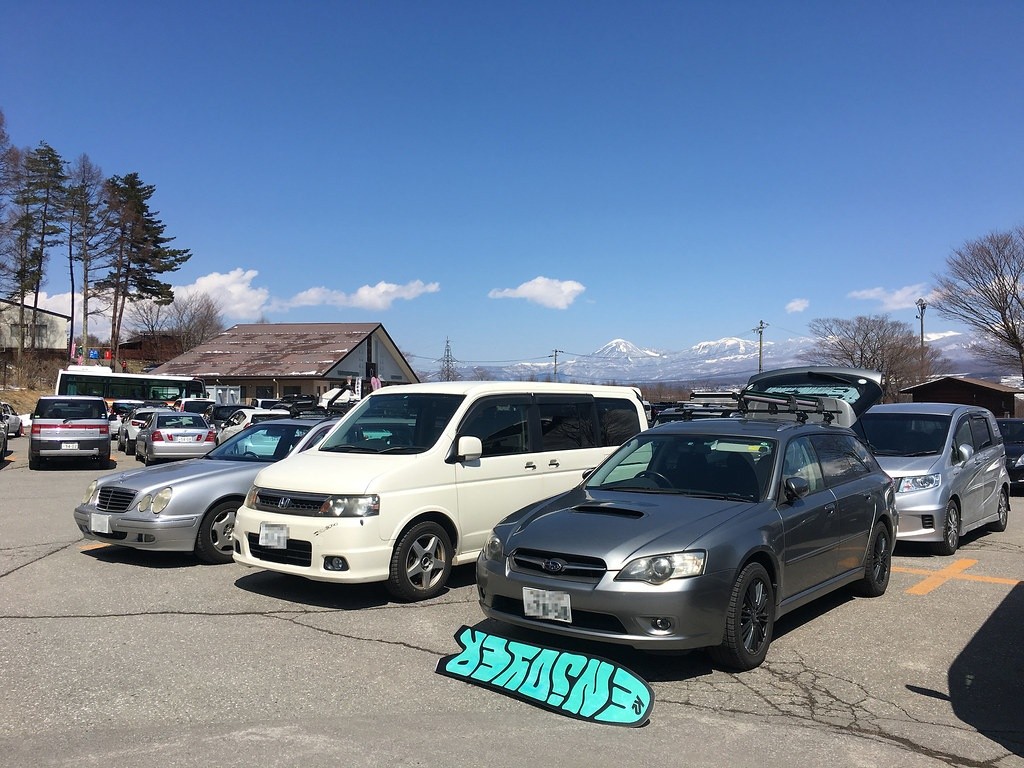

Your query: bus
(55, 367), (209, 411)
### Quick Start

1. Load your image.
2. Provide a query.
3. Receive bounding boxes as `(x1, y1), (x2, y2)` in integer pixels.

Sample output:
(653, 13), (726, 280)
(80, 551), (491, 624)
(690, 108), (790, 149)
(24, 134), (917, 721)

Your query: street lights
(916, 302), (927, 380)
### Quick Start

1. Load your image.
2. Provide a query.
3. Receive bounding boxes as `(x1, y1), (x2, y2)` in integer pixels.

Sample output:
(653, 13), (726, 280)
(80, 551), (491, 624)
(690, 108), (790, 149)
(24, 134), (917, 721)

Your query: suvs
(475, 365), (899, 669)
(28, 396), (110, 468)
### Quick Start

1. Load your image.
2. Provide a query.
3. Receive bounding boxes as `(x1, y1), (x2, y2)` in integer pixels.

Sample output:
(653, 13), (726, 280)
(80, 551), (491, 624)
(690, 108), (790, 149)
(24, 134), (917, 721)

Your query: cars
(850, 402), (1013, 554)
(204, 406), (249, 428)
(218, 407), (297, 456)
(251, 396), (381, 420)
(0, 402), (24, 465)
(73, 418), (416, 561)
(995, 419), (1024, 483)
(109, 400), (218, 463)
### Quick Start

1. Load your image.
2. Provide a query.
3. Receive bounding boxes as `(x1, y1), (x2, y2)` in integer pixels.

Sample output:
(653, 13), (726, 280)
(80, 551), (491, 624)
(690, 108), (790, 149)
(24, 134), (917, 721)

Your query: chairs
(481, 415), (522, 454)
(112, 388), (160, 399)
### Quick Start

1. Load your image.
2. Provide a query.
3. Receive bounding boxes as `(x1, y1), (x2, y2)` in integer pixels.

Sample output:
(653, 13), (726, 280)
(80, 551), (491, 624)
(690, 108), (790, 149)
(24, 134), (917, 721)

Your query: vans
(232, 380), (654, 601)
